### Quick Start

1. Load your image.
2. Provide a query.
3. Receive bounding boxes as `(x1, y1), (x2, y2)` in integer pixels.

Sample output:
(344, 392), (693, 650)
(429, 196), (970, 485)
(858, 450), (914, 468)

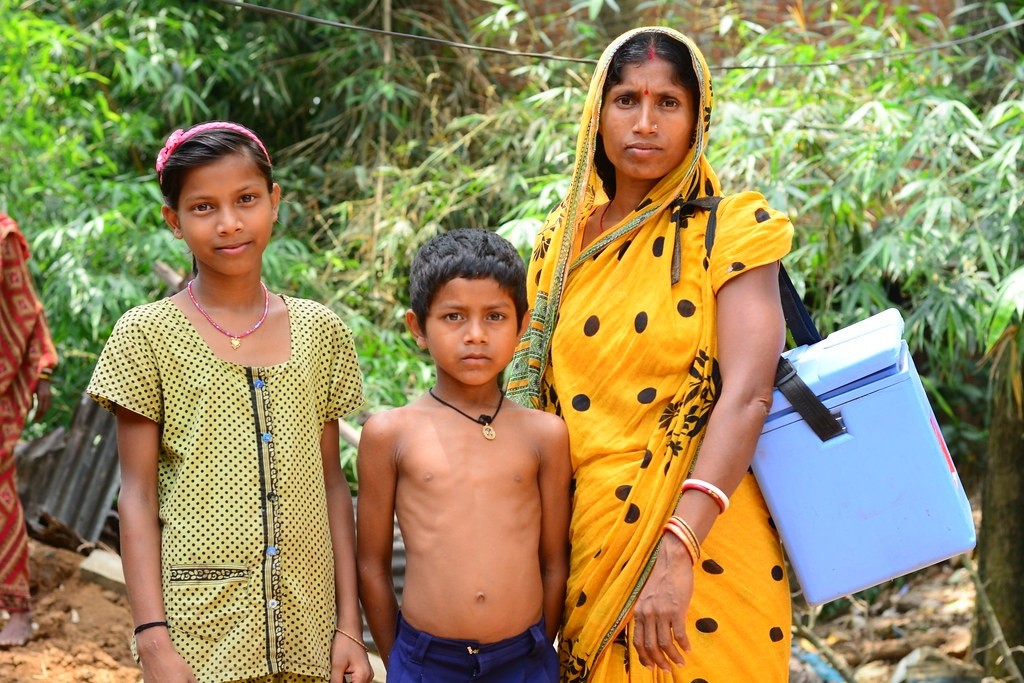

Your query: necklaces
(600, 200), (614, 233)
(429, 387), (505, 440)
(186, 278), (269, 351)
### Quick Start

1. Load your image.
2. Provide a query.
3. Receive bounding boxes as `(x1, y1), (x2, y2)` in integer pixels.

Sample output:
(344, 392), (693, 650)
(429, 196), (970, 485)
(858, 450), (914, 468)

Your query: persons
(0, 212), (60, 647)
(493, 23), (793, 683)
(355, 229), (571, 683)
(85, 121), (376, 683)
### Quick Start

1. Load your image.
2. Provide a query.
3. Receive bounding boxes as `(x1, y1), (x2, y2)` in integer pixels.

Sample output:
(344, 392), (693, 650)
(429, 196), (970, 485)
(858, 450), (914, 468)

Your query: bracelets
(335, 628), (370, 652)
(133, 620), (168, 635)
(40, 373), (50, 379)
(42, 367), (53, 374)
(663, 478), (729, 567)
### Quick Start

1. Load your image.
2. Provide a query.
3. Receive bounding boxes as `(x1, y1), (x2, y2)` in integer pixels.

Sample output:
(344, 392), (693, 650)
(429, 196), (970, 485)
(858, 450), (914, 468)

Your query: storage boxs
(750, 307), (976, 607)
